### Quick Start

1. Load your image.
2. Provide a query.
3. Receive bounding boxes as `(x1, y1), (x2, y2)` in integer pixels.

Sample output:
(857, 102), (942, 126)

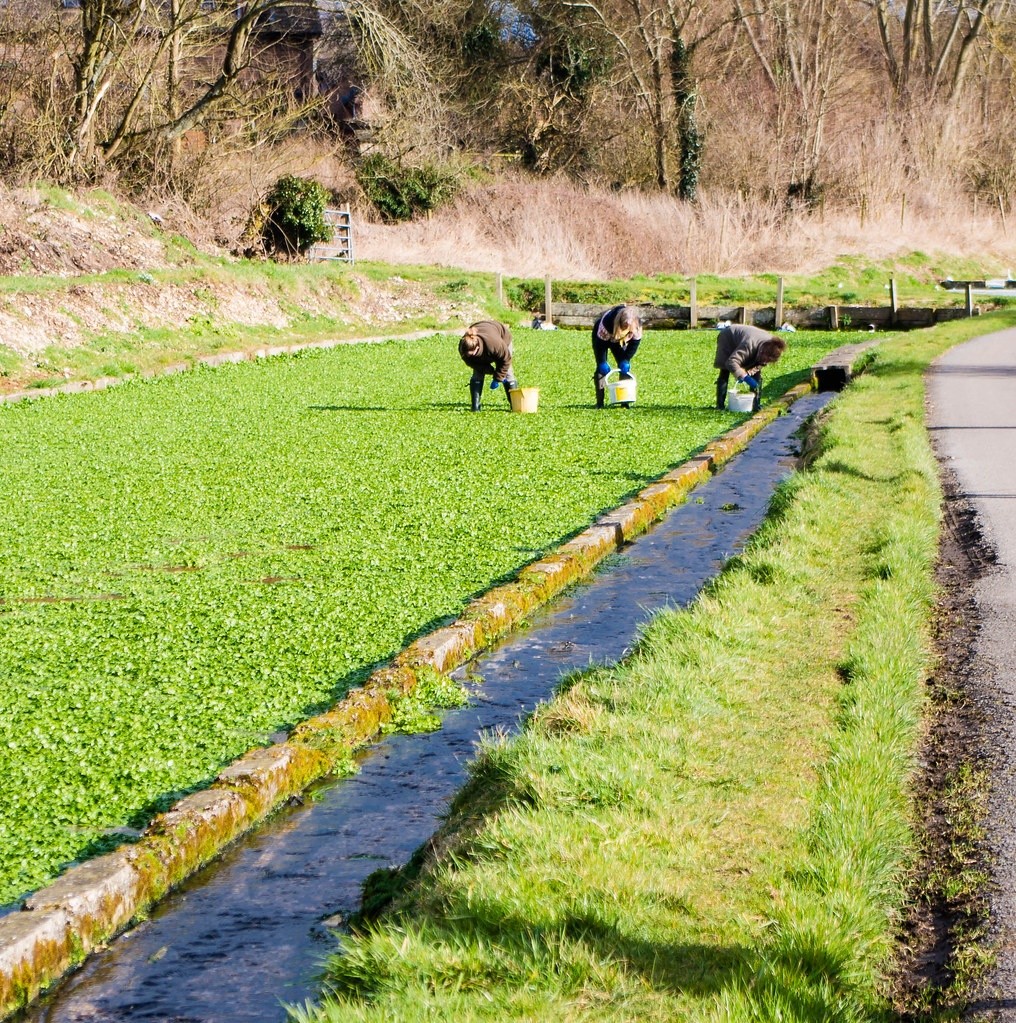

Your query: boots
(593, 373), (604, 409)
(717, 378), (729, 409)
(751, 377), (761, 412)
(619, 375), (630, 409)
(470, 379), (483, 411)
(504, 380), (517, 410)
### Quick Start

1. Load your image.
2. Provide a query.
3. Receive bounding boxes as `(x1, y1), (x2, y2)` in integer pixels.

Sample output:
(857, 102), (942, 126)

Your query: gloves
(490, 379), (499, 389)
(599, 361), (610, 376)
(738, 371), (750, 383)
(618, 359), (630, 375)
(743, 375), (758, 389)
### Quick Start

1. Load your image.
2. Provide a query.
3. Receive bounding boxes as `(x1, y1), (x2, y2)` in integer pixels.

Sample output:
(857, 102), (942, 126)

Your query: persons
(591, 304), (642, 408)
(714, 324), (787, 412)
(458, 320), (517, 412)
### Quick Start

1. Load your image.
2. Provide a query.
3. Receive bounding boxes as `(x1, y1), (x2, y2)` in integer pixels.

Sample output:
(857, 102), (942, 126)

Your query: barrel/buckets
(509, 387), (539, 413)
(726, 380), (756, 414)
(604, 369), (637, 404)
